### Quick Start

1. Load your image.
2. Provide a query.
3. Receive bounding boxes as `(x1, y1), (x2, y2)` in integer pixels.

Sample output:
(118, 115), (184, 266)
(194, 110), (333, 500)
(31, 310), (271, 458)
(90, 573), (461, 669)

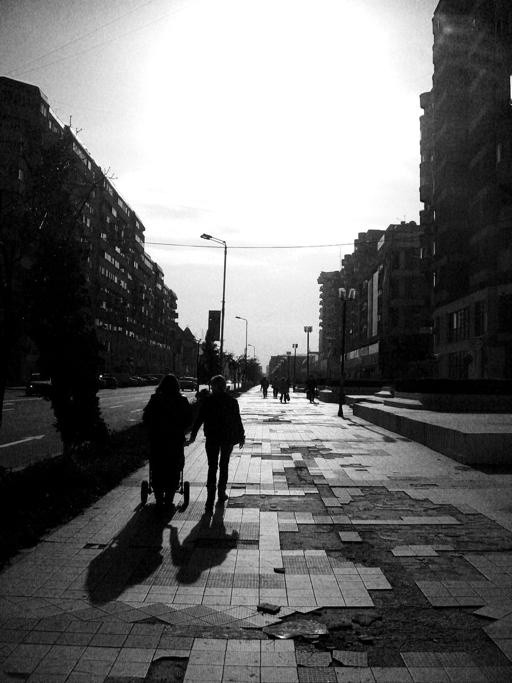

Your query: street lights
(270, 325), (312, 390)
(236, 316), (248, 361)
(200, 233), (227, 378)
(248, 344), (256, 361)
(338, 288), (356, 416)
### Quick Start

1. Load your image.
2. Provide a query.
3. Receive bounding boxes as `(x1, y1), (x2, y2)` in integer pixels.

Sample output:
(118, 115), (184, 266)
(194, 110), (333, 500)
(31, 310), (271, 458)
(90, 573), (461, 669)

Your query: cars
(28, 369), (198, 397)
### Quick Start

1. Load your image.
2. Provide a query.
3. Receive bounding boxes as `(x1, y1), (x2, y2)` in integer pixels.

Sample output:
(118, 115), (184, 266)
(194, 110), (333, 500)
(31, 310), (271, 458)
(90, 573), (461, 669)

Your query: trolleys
(140, 440), (190, 505)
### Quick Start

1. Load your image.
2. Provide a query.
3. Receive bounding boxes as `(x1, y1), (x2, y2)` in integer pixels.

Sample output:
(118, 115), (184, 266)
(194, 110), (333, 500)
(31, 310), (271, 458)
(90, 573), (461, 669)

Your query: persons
(191, 371), (247, 500)
(278, 377), (290, 403)
(271, 376), (279, 398)
(259, 376), (269, 398)
(305, 374), (318, 403)
(141, 372), (191, 513)
